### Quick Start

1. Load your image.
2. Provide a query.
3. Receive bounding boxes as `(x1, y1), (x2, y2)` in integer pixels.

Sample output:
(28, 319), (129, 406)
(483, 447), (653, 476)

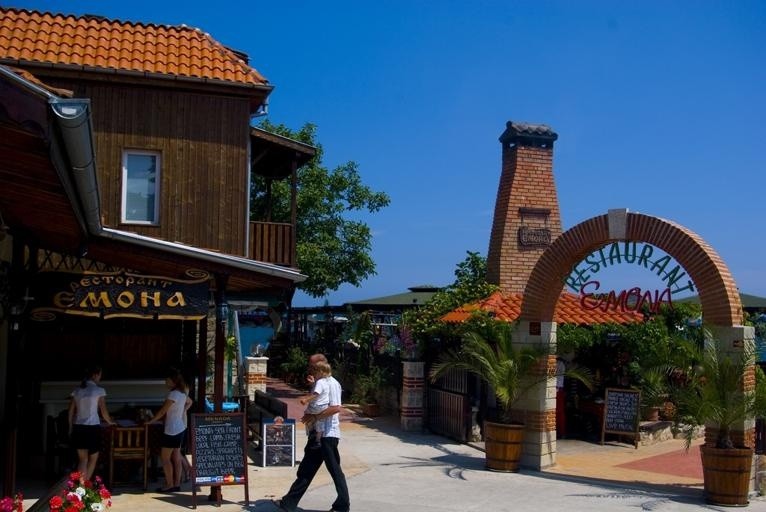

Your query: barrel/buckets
(699, 444), (752, 505)
(482, 419), (526, 472)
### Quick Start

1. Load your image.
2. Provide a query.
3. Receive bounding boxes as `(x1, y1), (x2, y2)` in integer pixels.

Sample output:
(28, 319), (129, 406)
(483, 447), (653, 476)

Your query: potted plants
(350, 365), (390, 418)
(429, 331), (599, 474)
(637, 321), (766, 507)
(630, 361), (660, 421)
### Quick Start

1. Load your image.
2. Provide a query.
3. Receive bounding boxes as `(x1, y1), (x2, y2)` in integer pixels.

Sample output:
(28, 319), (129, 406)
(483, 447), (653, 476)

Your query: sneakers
(155, 485), (181, 493)
(268, 498), (298, 512)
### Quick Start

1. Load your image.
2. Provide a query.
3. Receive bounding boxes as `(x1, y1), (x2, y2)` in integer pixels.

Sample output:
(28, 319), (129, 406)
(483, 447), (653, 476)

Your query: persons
(67, 365), (120, 482)
(556, 353), (568, 440)
(269, 352), (351, 512)
(300, 360), (331, 449)
(178, 374), (194, 484)
(143, 368), (194, 493)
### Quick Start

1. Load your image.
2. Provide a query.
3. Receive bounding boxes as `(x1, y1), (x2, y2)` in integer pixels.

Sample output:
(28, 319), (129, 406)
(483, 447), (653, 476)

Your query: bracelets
(314, 415), (318, 421)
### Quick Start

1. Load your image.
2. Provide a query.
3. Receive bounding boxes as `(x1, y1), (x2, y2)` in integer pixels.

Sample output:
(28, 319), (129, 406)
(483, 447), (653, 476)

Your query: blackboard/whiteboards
(262, 420), (294, 468)
(603, 388), (641, 435)
(191, 412), (248, 486)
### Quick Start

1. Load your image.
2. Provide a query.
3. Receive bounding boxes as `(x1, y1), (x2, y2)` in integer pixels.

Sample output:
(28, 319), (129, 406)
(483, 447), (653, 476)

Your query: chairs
(109, 417), (156, 490)
